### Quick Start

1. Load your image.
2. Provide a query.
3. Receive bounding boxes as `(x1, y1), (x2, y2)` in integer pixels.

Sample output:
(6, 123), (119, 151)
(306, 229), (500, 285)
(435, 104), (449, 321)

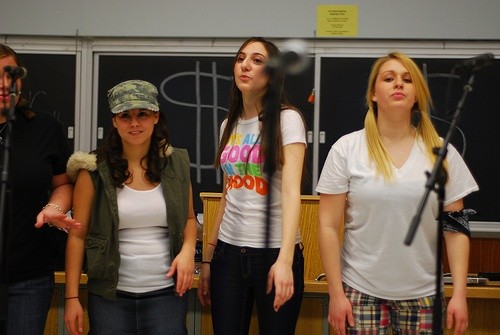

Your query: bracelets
(208, 242), (217, 248)
(201, 261), (211, 264)
(65, 296), (79, 300)
(42, 204), (64, 214)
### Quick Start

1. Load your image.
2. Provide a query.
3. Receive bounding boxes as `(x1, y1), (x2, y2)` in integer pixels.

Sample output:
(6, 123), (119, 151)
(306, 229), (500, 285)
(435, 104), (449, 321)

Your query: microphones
(464, 53), (496, 73)
(4, 66), (27, 79)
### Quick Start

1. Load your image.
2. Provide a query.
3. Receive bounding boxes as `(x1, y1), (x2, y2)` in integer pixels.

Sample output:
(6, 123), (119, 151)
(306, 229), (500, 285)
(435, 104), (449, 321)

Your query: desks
(44, 268), (500, 335)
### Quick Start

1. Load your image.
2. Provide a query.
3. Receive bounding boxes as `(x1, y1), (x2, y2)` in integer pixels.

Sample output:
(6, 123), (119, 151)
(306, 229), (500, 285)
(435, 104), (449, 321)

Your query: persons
(64, 78), (198, 335)
(315, 52), (479, 335)
(197, 37), (306, 335)
(0, 43), (82, 335)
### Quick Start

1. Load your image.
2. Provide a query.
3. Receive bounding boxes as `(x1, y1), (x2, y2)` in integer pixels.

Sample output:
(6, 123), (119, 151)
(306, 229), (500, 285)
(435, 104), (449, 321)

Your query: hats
(107, 80), (159, 114)
